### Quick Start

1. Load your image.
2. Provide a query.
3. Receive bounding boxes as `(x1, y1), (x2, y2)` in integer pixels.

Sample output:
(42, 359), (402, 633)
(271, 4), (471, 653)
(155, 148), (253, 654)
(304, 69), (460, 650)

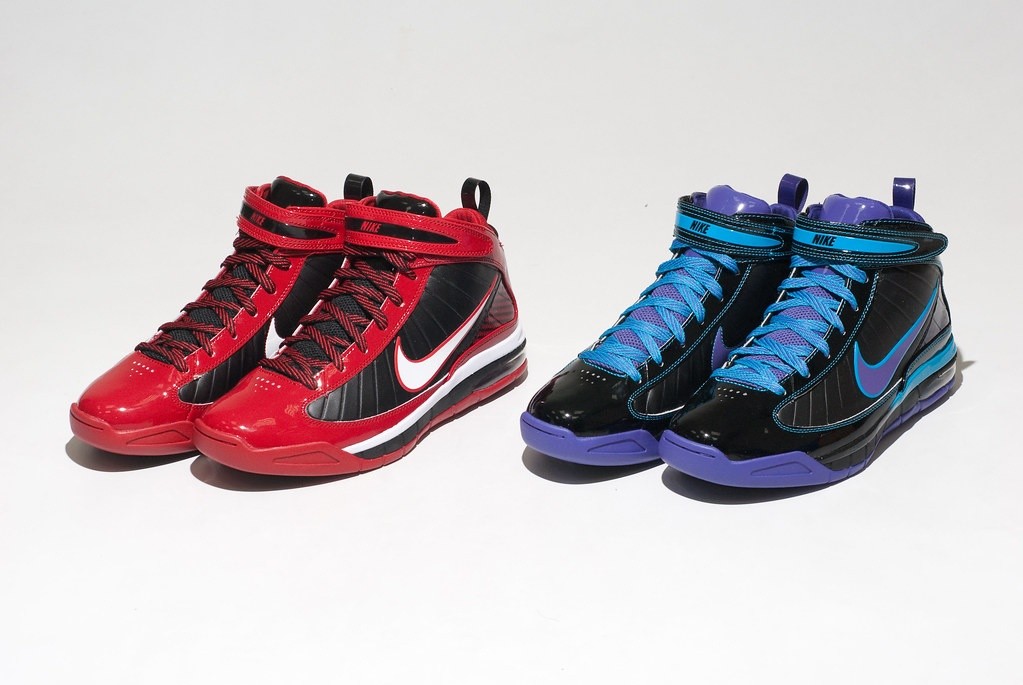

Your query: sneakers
(658, 172), (957, 493)
(69, 173), (380, 457)
(518, 172), (809, 465)
(192, 175), (529, 478)
